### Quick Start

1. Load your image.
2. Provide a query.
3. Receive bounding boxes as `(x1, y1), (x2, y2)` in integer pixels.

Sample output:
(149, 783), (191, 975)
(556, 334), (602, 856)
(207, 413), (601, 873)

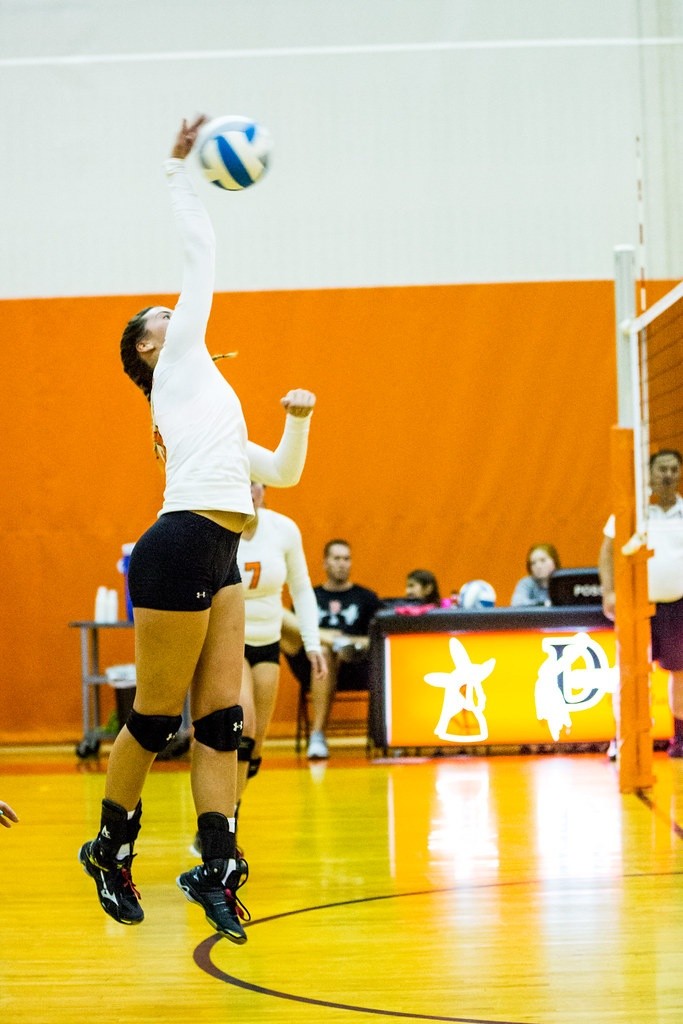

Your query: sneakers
(178, 865), (251, 945)
(78, 840), (145, 925)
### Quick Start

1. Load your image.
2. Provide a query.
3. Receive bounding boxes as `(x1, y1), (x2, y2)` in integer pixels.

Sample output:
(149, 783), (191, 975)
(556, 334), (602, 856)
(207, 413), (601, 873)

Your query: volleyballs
(187, 111), (277, 195)
(456, 577), (499, 613)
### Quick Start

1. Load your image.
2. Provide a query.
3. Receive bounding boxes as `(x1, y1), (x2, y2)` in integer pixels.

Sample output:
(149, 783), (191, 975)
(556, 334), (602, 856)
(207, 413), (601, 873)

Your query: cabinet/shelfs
(73, 622), (196, 749)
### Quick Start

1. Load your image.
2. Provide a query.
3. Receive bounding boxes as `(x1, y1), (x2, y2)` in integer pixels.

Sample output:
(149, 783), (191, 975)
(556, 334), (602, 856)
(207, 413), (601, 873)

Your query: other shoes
(190, 832), (244, 864)
(668, 743), (682, 757)
(306, 731), (330, 760)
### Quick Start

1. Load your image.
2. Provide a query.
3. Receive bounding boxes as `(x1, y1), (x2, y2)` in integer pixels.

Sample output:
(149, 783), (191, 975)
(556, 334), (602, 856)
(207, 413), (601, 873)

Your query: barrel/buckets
(117, 542), (135, 622)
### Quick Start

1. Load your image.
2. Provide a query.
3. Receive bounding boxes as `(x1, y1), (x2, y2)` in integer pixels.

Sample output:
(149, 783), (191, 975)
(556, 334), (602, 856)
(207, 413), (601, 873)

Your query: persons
(0, 800), (19, 828)
(281, 538), (384, 759)
(188, 474), (327, 874)
(597, 447), (683, 759)
(509, 541), (561, 607)
(403, 568), (442, 607)
(77, 110), (319, 945)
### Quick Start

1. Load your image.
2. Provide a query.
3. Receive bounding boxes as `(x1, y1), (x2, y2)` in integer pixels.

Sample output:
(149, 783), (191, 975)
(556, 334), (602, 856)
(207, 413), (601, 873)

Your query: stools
(296, 683), (374, 760)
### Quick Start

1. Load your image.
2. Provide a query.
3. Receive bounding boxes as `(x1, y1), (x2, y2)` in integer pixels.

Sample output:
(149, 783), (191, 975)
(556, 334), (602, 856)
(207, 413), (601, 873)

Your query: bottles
(95, 587), (107, 623)
(107, 590), (118, 623)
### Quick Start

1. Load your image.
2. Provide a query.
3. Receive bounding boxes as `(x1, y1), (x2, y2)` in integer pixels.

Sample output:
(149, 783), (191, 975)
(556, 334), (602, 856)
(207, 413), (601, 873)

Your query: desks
(373, 604), (617, 749)
